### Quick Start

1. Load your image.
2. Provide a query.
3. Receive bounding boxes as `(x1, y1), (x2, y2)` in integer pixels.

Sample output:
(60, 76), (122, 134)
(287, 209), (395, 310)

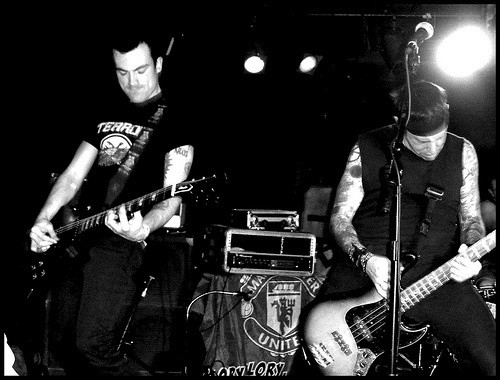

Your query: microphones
(405, 21), (434, 54)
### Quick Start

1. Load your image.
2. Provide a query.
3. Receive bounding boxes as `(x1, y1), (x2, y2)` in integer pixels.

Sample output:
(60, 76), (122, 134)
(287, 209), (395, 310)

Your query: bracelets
(348, 243), (376, 273)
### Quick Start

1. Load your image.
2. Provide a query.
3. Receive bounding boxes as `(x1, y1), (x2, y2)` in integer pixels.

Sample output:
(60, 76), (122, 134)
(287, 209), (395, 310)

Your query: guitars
(23, 173), (232, 298)
(303, 229), (496, 376)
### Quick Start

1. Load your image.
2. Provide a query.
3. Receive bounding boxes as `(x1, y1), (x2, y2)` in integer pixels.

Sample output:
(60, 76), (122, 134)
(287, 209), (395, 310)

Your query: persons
(304, 80), (496, 376)
(30, 33), (200, 380)
